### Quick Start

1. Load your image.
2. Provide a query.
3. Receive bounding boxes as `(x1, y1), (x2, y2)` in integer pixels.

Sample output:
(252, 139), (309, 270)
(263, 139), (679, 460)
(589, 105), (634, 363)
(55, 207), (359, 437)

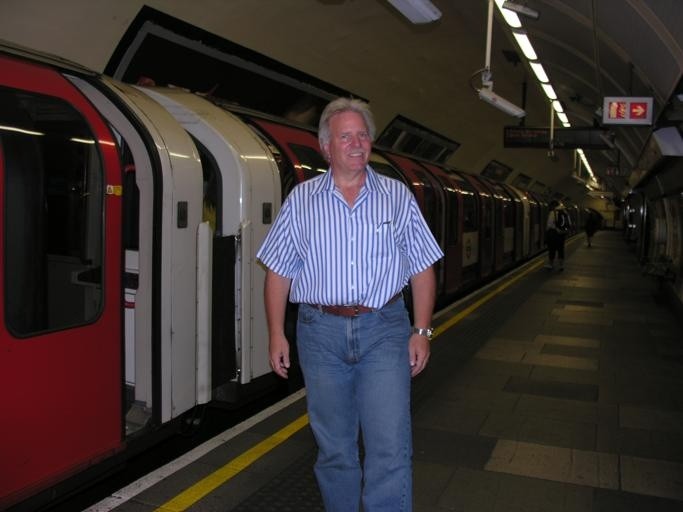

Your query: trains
(0, 56), (607, 511)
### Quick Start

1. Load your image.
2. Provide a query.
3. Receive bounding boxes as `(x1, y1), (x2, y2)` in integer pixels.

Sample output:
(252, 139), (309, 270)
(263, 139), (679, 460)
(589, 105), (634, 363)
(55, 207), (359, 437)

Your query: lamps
(475, 0), (571, 127)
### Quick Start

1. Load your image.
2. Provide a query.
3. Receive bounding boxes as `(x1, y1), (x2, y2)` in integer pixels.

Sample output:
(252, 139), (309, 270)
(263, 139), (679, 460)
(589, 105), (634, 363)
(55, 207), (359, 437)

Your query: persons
(255, 98), (447, 511)
(541, 199), (597, 272)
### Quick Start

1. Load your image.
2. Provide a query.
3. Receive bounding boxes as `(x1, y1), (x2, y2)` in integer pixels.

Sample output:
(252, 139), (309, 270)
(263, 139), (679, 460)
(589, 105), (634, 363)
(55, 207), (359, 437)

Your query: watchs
(412, 327), (437, 341)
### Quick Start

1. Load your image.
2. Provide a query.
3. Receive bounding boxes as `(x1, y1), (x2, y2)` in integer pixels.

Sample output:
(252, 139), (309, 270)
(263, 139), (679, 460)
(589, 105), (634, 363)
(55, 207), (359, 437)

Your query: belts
(309, 293), (402, 317)
